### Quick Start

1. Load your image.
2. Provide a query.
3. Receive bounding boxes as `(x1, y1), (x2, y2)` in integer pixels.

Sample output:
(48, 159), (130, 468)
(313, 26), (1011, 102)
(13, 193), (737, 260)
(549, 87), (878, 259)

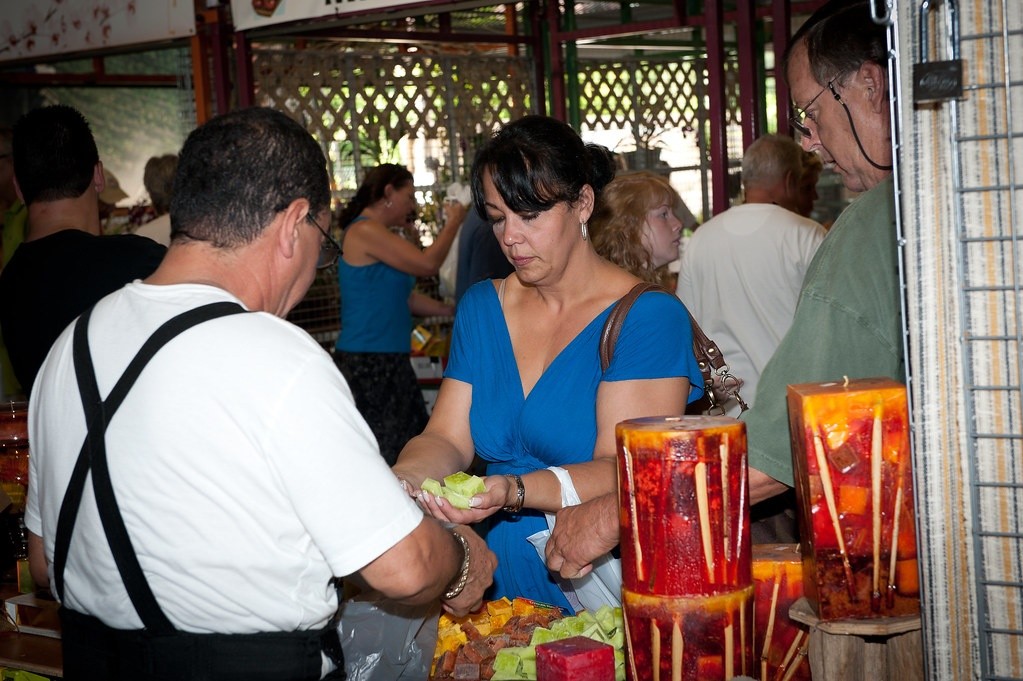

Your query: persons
(132, 154), (182, 249)
(592, 171), (681, 289)
(389, 113), (703, 619)
(23, 106), (497, 681)
(671, 137), (836, 414)
(332, 164), (472, 467)
(454, 204), (518, 304)
(0, 107), (166, 401)
(541, 0), (911, 578)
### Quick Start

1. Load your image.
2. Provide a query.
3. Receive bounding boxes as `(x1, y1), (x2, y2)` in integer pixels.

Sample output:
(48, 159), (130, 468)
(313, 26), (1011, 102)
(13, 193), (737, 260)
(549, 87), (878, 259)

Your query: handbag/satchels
(526, 466), (622, 615)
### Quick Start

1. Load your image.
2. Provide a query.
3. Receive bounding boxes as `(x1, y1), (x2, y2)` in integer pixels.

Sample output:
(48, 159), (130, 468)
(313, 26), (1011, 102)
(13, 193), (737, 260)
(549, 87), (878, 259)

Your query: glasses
(788, 56), (877, 139)
(275, 204), (344, 268)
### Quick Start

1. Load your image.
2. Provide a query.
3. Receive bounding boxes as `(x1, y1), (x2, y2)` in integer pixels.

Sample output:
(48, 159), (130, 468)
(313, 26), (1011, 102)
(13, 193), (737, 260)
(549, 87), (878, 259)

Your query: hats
(97, 168), (129, 205)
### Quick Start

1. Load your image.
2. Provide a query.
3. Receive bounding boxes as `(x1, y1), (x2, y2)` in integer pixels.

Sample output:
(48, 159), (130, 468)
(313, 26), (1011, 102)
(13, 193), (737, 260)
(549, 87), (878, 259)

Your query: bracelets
(503, 473), (526, 514)
(445, 533), (471, 601)
